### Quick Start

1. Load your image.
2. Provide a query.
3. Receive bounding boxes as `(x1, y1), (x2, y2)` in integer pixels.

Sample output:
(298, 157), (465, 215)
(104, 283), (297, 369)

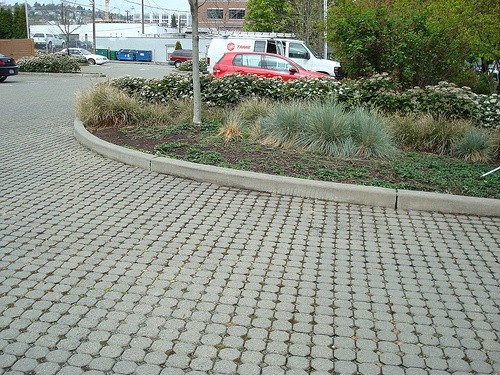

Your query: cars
(0, 52), (19, 83)
(55, 47), (109, 65)
(212, 52), (331, 83)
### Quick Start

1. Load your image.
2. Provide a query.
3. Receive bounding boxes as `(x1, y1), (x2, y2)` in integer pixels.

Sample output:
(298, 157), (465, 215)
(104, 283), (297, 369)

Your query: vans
(206, 31), (342, 77)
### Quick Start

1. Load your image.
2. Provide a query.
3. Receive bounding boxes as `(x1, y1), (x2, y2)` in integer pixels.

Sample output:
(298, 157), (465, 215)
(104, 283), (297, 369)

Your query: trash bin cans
(95, 48), (109, 59)
(116, 49), (135, 61)
(110, 50), (117, 60)
(135, 50), (153, 61)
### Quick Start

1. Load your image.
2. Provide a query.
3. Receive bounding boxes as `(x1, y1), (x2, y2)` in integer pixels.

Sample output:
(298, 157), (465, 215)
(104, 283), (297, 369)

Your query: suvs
(33, 32), (66, 49)
(168, 49), (194, 68)
